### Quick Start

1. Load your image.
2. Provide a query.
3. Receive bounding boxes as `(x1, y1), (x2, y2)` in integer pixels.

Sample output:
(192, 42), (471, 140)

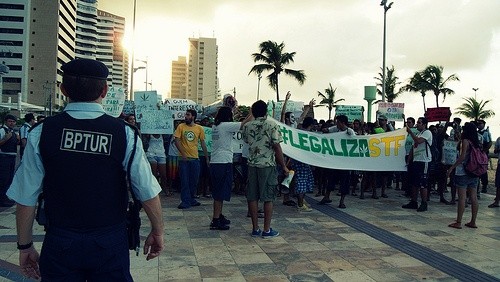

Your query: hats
(60, 58), (108, 80)
(378, 114), (388, 119)
(5, 113), (17, 121)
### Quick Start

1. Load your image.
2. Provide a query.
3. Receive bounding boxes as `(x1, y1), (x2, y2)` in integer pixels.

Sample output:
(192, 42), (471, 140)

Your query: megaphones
(276, 170), (295, 194)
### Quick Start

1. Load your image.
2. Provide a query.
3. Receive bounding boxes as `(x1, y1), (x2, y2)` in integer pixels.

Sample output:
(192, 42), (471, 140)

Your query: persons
(118, 92), (492, 212)
(488, 137), (500, 208)
(173, 109), (210, 209)
(6, 59), (163, 282)
(210, 107), (252, 230)
(19, 113), (36, 161)
(447, 125), (479, 229)
(242, 100), (289, 238)
(0, 114), (20, 207)
(37, 116), (45, 123)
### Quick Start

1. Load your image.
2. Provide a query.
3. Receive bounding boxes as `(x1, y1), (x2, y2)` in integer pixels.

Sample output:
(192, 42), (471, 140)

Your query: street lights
(473, 88), (478, 101)
(380, 0), (394, 105)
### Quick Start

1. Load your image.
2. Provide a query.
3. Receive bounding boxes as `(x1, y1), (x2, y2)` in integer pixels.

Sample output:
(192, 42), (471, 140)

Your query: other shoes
(194, 193), (211, 198)
(488, 203), (500, 208)
(315, 192), (358, 208)
(248, 209), (265, 218)
(440, 198), (456, 205)
(283, 199), (312, 212)
(351, 192), (388, 200)
(481, 186), (487, 193)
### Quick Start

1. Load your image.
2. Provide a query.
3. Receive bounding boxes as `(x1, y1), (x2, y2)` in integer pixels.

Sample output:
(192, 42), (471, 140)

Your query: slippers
(449, 222), (461, 228)
(465, 221), (477, 228)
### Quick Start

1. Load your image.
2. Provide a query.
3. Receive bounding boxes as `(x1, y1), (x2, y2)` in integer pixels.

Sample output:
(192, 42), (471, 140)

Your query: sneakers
(251, 227), (279, 239)
(209, 213), (231, 230)
(402, 200), (427, 211)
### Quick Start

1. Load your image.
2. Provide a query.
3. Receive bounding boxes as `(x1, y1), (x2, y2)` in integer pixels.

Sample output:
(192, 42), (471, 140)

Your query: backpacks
(464, 139), (488, 178)
(430, 134), (440, 159)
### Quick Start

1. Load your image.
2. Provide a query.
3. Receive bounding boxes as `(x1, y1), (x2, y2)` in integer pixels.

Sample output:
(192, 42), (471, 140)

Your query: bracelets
(17, 241), (33, 250)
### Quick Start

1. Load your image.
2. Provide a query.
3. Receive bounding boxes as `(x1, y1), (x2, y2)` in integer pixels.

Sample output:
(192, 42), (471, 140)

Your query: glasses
(417, 121), (421, 124)
(352, 123), (359, 125)
